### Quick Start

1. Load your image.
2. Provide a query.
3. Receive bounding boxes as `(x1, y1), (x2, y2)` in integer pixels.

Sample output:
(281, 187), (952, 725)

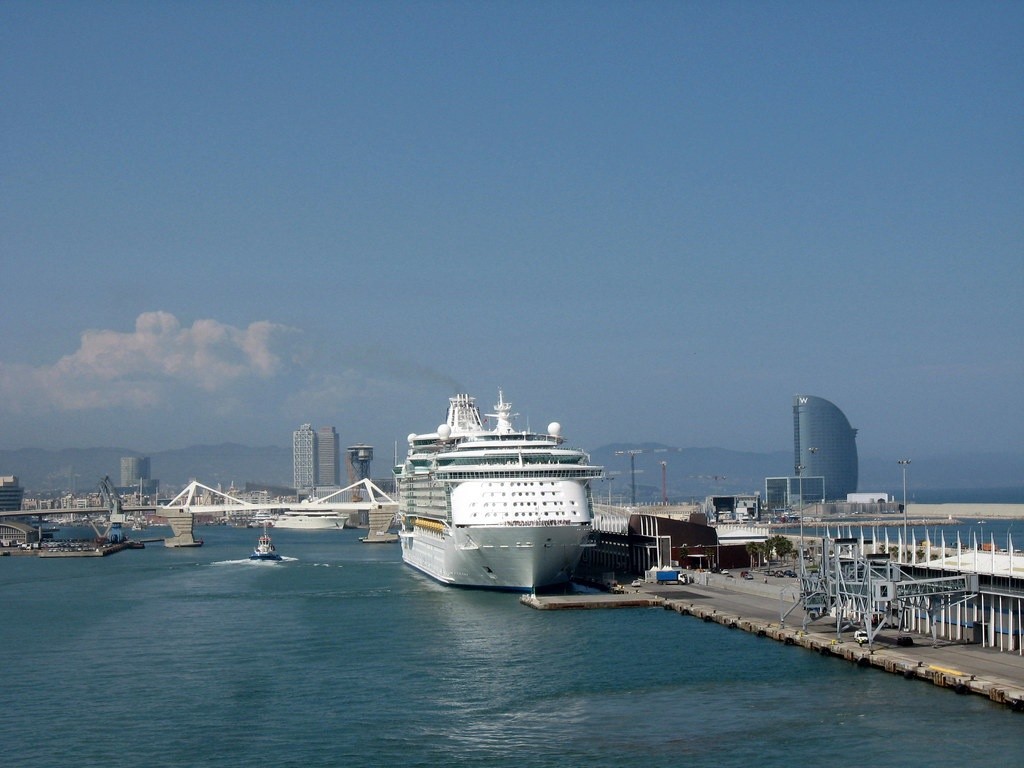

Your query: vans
(854, 631), (869, 643)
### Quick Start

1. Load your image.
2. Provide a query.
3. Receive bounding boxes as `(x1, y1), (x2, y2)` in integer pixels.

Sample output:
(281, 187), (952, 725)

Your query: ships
(392, 384), (606, 594)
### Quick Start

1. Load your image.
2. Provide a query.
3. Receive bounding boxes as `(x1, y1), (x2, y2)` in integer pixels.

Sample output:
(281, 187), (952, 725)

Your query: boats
(249, 520), (283, 560)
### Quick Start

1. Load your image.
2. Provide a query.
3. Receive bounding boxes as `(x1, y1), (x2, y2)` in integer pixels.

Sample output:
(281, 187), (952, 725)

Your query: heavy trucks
(655, 570), (687, 585)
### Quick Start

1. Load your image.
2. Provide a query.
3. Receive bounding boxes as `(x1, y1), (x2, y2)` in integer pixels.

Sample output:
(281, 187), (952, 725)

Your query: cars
(897, 637), (913, 647)
(631, 580), (640, 587)
(789, 572), (797, 578)
(785, 570), (792, 575)
(42, 538), (96, 552)
(721, 569), (729, 575)
(740, 571), (748, 577)
(775, 571), (784, 577)
(744, 573), (753, 580)
